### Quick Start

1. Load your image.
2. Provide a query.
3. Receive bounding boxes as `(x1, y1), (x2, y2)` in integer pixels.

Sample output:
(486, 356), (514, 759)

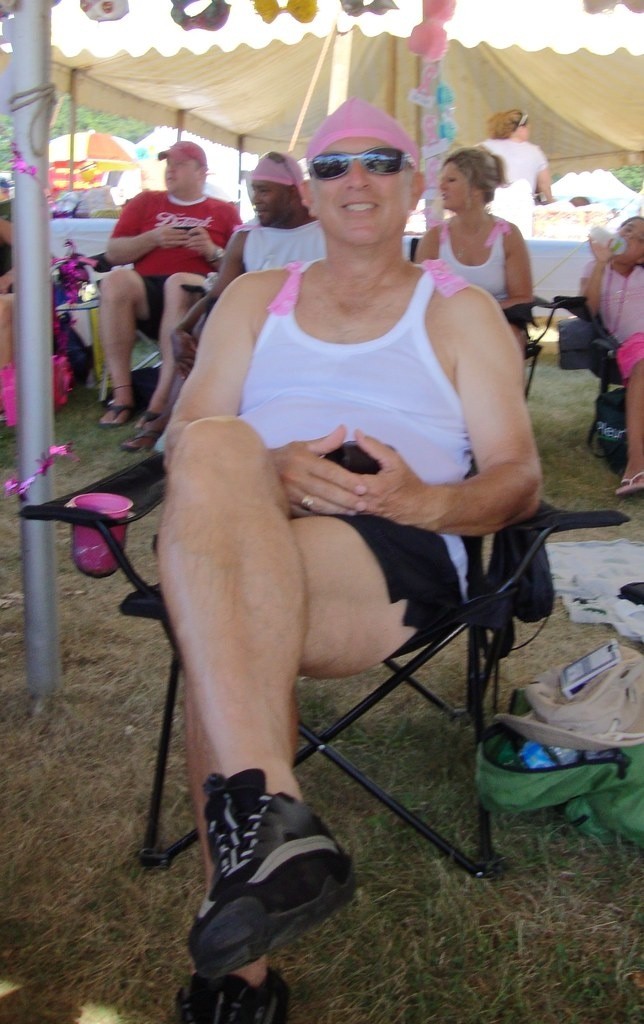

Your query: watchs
(207, 247), (224, 262)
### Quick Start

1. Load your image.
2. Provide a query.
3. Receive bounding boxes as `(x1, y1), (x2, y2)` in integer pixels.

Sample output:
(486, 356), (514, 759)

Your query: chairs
(555, 293), (624, 448)
(95, 253), (208, 403)
(21, 339), (628, 877)
(404, 237), (559, 398)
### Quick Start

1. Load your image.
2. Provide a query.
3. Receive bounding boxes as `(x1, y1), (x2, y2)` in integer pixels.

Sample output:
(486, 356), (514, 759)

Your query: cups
(71, 492), (134, 574)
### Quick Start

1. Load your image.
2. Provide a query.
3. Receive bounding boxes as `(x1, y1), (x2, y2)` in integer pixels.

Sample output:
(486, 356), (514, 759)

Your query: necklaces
(605, 261), (628, 335)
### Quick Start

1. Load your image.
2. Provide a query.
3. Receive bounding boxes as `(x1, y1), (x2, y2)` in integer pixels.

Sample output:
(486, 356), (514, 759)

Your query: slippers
(136, 409), (160, 429)
(120, 428), (159, 451)
(99, 402), (137, 428)
(614, 473), (644, 496)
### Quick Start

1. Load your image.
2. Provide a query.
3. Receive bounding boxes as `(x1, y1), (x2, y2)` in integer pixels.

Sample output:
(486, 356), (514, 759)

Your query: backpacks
(473, 649), (644, 841)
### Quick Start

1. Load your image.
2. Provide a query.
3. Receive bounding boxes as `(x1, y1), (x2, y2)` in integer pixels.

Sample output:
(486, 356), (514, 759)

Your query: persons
(580, 216), (644, 497)
(155, 96), (543, 1024)
(477, 109), (555, 239)
(0, 174), (16, 422)
(413, 143), (534, 353)
(121, 148), (326, 452)
(97, 141), (243, 451)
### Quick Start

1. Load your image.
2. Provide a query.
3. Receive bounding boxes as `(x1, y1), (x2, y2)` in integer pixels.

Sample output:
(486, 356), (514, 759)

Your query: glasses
(309, 151), (411, 178)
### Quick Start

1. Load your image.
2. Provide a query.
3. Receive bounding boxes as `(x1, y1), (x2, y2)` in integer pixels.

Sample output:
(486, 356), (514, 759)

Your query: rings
(300, 493), (319, 509)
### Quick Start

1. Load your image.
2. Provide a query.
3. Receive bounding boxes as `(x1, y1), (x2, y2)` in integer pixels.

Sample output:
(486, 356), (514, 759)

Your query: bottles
(591, 227), (627, 255)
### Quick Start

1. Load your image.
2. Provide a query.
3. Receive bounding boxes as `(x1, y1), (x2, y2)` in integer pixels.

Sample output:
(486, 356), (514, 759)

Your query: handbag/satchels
(586, 388), (629, 473)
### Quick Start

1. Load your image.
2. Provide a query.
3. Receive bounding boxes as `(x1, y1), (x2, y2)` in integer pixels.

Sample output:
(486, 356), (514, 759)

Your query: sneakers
(185, 769), (358, 975)
(177, 966), (286, 1024)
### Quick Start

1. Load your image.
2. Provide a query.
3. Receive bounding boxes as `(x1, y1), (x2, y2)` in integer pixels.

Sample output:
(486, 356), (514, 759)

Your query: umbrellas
(0, 0), (644, 135)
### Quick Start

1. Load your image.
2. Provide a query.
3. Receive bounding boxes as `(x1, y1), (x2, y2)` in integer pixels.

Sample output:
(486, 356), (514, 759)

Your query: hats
(308, 102), (421, 172)
(158, 141), (206, 168)
